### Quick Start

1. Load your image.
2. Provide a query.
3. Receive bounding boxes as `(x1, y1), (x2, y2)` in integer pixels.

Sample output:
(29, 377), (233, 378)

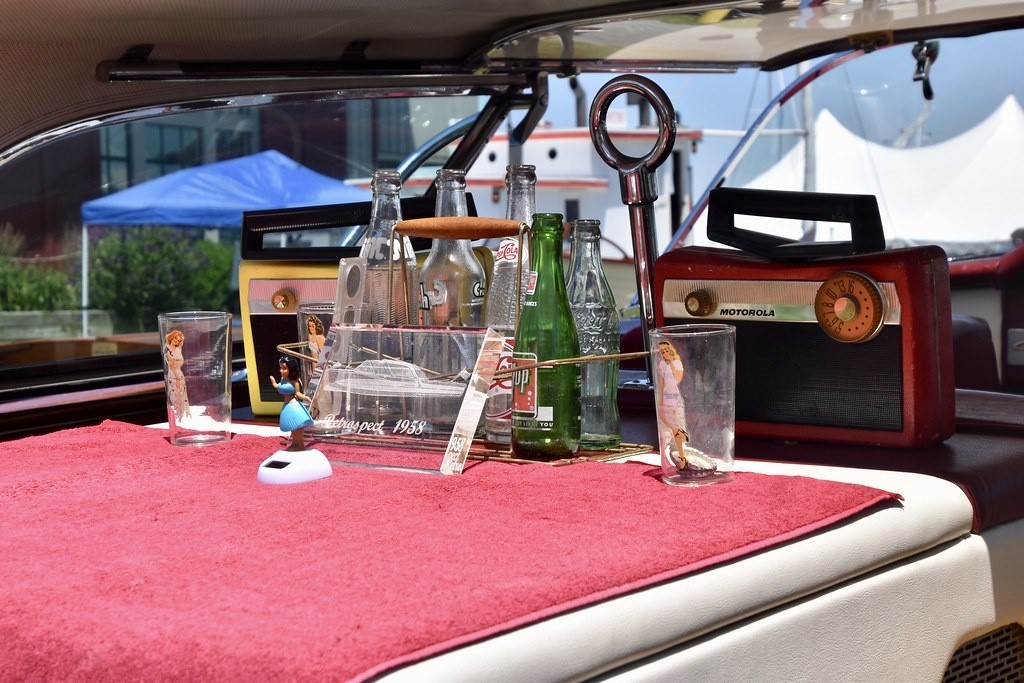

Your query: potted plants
(0, 264), (119, 343)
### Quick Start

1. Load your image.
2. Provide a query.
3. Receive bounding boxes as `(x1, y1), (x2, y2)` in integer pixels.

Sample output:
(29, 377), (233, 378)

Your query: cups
(158, 311), (233, 446)
(296, 304), (359, 415)
(649, 324), (737, 487)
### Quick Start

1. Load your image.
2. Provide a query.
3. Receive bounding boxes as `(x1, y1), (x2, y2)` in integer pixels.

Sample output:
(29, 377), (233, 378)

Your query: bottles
(566, 219), (623, 451)
(356, 170), (418, 430)
(415, 170), (487, 436)
(483, 165), (538, 444)
(513, 213), (581, 461)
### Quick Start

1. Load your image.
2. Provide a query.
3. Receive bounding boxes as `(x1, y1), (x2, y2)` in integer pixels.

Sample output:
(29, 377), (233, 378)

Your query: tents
(80, 149), (373, 337)
(601, 96), (1024, 258)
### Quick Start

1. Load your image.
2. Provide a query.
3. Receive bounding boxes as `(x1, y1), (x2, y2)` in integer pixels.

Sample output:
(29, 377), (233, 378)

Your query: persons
(270, 355), (312, 451)
